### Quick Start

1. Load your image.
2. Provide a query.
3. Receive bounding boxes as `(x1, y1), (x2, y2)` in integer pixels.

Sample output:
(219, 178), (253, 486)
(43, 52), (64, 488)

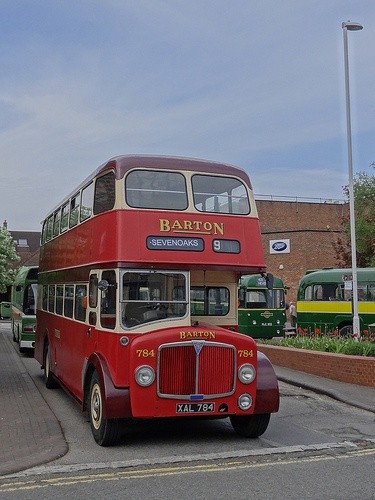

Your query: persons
(289, 302), (296, 328)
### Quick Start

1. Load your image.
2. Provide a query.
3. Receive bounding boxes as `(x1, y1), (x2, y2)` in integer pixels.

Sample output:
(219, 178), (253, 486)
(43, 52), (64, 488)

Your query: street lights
(341, 19), (363, 340)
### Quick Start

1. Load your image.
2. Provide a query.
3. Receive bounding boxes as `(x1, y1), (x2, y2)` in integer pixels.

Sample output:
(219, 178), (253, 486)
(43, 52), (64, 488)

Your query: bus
(11, 265), (39, 356)
(34, 153), (280, 446)
(296, 267), (375, 344)
(191, 272), (289, 339)
